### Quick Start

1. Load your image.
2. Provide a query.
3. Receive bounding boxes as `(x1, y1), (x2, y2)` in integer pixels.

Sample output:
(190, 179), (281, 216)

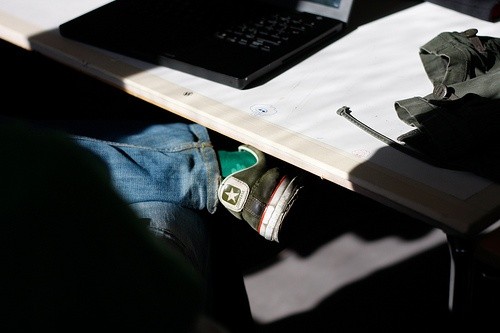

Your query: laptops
(59, 0), (354, 91)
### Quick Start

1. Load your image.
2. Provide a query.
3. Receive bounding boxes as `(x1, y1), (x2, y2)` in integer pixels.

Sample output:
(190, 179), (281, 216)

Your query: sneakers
(216, 143), (310, 244)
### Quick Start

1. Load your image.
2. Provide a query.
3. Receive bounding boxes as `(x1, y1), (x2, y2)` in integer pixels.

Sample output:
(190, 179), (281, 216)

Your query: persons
(0, 122), (307, 333)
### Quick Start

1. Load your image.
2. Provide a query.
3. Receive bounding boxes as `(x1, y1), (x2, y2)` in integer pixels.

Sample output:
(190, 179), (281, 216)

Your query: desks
(0, 0), (500, 333)
